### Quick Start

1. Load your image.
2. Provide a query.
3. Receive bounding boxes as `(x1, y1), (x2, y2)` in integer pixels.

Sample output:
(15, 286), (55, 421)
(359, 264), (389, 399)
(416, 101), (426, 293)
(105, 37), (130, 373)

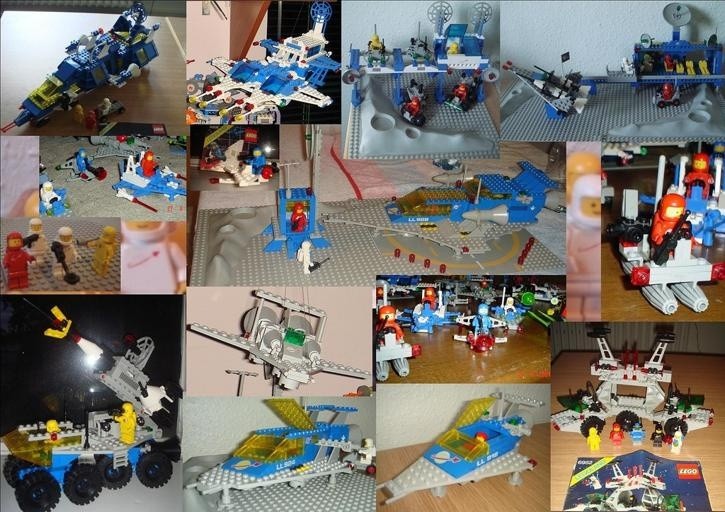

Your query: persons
(1, 1), (725, 510)
(0, 0), (725, 512)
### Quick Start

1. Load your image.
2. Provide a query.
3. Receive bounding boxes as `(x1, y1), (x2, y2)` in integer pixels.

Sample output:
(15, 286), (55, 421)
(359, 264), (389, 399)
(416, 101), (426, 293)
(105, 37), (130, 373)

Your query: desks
(551, 348), (724, 511)
(1, 11), (191, 137)
(376, 422), (551, 512)
